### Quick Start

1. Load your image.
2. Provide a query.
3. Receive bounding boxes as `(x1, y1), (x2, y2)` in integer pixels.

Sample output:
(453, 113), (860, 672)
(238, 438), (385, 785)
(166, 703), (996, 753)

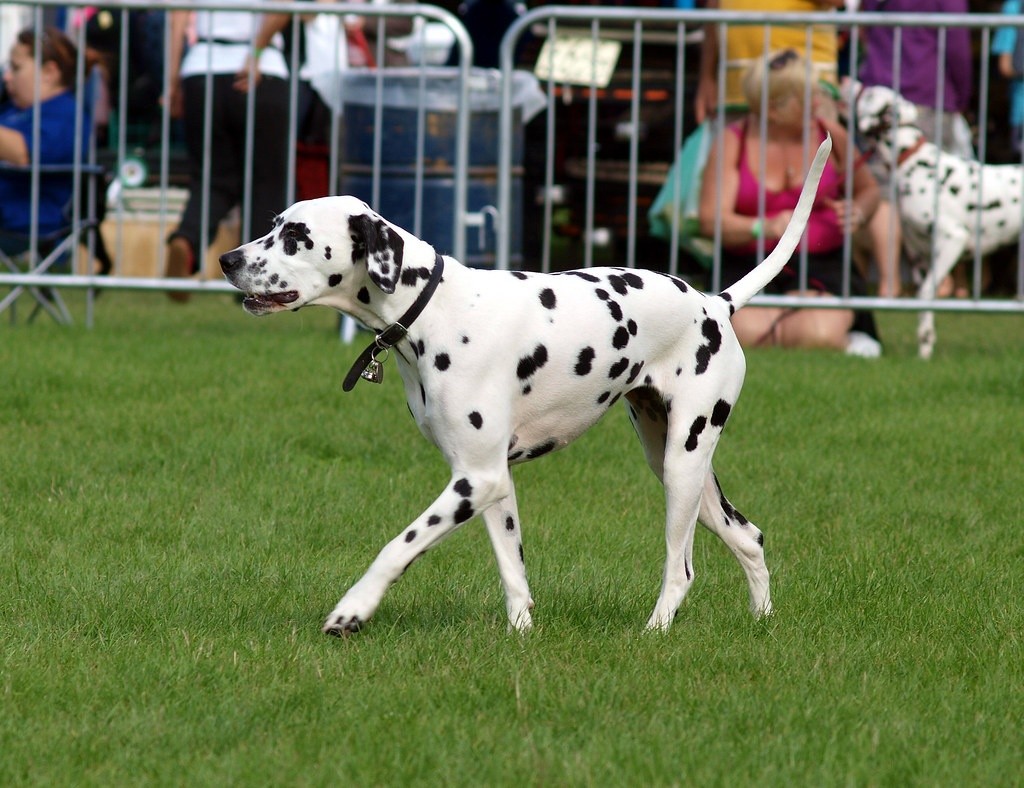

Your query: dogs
(839, 77), (1024, 362)
(218, 130), (833, 641)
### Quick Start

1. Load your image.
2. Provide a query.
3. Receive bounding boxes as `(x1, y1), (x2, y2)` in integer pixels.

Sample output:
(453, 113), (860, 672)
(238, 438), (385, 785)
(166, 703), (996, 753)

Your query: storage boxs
(341, 65), (526, 265)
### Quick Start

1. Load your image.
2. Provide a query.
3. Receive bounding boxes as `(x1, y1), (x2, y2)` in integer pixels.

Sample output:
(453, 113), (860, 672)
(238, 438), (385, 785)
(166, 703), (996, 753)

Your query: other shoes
(166, 237), (194, 304)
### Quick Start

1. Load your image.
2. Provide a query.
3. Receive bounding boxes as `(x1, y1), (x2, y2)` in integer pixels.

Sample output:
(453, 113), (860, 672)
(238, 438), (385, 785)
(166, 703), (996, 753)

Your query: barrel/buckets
(335, 66), (524, 278)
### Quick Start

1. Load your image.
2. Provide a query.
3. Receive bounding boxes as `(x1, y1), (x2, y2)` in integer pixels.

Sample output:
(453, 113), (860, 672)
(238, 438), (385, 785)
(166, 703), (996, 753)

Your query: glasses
(769, 50), (799, 70)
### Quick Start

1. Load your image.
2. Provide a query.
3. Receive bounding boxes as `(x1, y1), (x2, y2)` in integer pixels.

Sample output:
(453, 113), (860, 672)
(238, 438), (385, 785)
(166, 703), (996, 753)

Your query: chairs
(0, 64), (107, 328)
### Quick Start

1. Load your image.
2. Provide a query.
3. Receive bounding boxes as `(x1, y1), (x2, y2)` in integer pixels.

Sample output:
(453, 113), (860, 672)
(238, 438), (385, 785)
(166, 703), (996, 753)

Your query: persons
(698, 50), (884, 360)
(990, 0), (1024, 156)
(161, 9), (290, 304)
(859, 0), (972, 167)
(293, 13), (374, 145)
(0, 28), (91, 258)
(696, 0), (843, 125)
